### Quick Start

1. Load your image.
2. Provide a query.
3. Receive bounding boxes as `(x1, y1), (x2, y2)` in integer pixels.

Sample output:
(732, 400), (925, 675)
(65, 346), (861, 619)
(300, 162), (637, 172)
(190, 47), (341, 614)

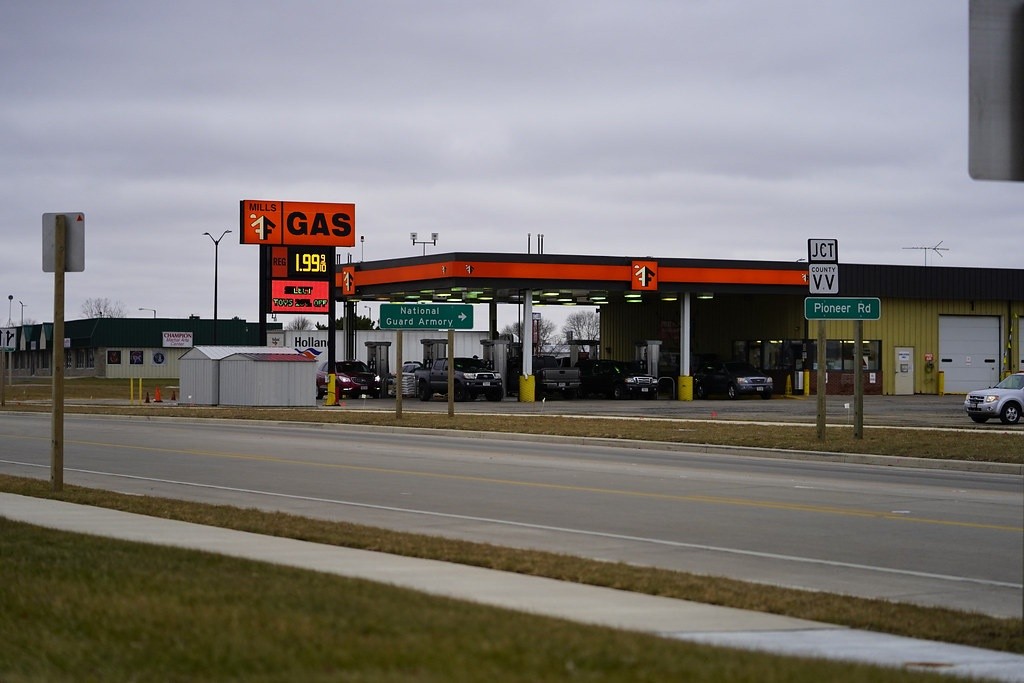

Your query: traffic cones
(144, 392), (151, 403)
(154, 384), (163, 402)
(171, 390), (176, 400)
(783, 373), (793, 396)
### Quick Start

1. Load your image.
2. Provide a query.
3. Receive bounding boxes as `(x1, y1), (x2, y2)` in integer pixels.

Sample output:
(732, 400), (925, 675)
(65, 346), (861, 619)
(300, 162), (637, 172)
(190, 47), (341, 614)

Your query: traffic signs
(0, 328), (17, 352)
(380, 303), (474, 330)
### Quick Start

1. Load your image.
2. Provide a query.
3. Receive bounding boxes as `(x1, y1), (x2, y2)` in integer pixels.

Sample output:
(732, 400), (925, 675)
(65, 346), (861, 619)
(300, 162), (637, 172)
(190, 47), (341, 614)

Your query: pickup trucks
(415, 357), (504, 402)
(507, 355), (582, 399)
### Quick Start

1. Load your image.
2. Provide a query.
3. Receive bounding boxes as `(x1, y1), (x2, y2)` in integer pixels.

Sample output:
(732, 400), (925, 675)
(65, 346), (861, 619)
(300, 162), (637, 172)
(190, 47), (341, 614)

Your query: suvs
(574, 360), (659, 401)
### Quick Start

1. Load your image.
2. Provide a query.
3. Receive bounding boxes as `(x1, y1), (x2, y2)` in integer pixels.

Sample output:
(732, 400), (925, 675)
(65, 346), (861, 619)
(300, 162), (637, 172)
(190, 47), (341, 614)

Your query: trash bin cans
(677, 375), (693, 402)
(519, 375), (536, 402)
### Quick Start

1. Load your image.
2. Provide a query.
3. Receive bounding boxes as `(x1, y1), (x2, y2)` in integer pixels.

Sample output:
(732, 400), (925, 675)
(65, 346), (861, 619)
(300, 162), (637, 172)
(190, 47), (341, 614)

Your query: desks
(164, 386), (179, 400)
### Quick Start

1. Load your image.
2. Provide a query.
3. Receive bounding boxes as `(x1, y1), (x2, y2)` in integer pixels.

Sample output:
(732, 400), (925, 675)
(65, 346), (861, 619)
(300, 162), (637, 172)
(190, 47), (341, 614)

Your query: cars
(316, 359), (382, 399)
(963, 372), (1024, 425)
(693, 361), (774, 399)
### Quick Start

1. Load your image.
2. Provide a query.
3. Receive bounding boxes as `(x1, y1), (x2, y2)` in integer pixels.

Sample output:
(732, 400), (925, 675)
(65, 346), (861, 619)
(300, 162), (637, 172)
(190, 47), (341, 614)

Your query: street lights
(364, 306), (371, 319)
(139, 308), (156, 318)
(6, 295), (14, 327)
(203, 230), (231, 345)
(19, 301), (27, 326)
